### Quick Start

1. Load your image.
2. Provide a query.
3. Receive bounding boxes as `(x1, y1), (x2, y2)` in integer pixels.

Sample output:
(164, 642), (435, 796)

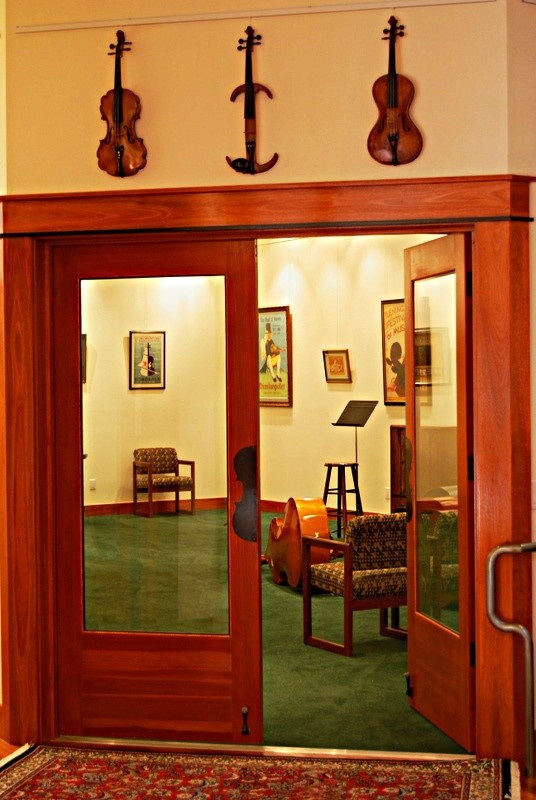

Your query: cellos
(260, 497), (331, 590)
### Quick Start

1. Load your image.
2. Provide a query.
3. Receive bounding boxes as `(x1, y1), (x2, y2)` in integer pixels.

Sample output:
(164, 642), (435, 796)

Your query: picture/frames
(128, 329), (167, 391)
(321, 349), (354, 385)
(259, 303), (293, 408)
(381, 299), (433, 406)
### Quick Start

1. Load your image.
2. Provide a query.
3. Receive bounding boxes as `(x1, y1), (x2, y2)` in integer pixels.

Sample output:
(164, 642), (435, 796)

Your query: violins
(367, 16), (423, 166)
(97, 29), (148, 178)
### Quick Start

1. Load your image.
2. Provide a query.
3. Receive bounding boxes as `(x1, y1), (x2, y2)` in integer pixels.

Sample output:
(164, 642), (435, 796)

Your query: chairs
(131, 447), (195, 519)
(301, 511), (460, 660)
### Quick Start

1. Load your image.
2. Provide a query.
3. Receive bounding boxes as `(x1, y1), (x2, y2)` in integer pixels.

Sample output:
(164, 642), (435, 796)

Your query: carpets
(0, 744), (512, 800)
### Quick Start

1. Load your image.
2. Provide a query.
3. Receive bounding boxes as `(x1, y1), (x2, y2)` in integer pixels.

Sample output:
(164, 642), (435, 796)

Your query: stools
(322, 462), (363, 539)
